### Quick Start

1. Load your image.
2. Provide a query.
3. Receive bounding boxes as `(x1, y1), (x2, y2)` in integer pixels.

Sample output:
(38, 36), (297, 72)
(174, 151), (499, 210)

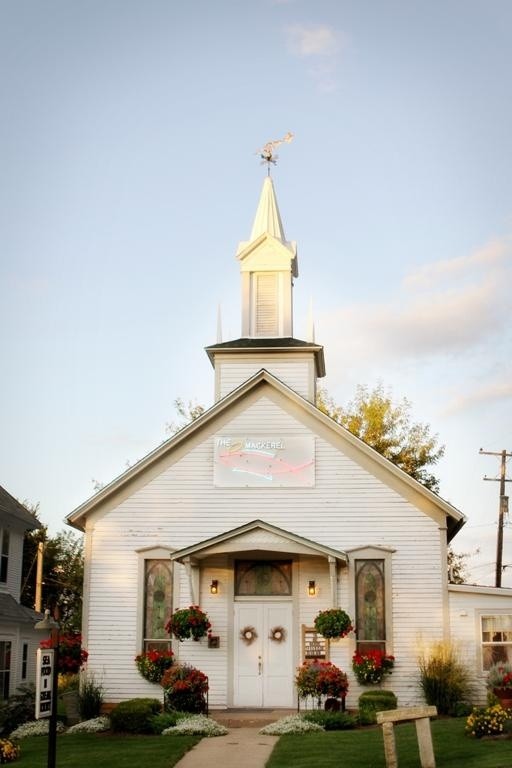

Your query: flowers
(486, 661), (512, 690)
(39, 633), (89, 674)
(291, 607), (393, 706)
(134, 605), (212, 713)
